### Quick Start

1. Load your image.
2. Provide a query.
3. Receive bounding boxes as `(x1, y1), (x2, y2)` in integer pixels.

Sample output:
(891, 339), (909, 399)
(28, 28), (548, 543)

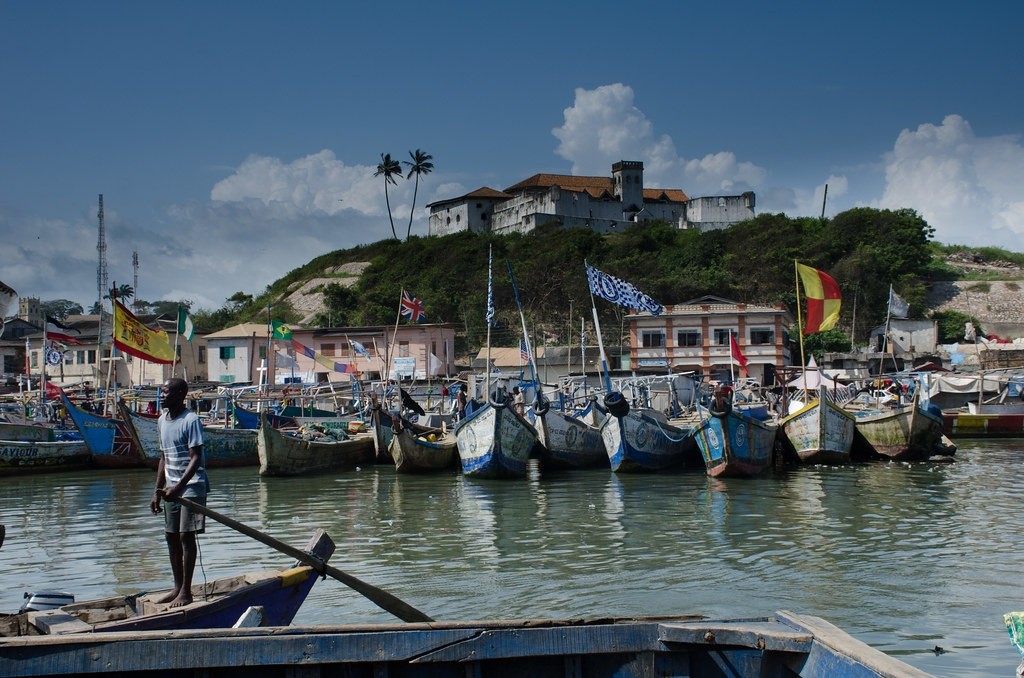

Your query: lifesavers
(488, 390), (510, 409)
(532, 398), (549, 416)
(604, 391), (630, 417)
(709, 397), (731, 418)
(390, 423), (404, 435)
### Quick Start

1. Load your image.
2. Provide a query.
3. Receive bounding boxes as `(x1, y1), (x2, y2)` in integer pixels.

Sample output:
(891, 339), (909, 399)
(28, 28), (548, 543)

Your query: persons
(54, 406), (59, 418)
(458, 384), (467, 420)
(503, 386), (525, 416)
(442, 382), (449, 396)
(92, 402), (103, 415)
(150, 378), (211, 608)
(83, 381), (89, 397)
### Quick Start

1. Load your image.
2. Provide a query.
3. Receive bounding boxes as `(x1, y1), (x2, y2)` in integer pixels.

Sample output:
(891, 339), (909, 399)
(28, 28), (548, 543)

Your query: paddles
(153, 486), (438, 623)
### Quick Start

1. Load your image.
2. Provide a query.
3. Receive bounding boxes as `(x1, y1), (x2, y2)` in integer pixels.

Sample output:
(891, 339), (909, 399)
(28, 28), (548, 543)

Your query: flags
(731, 334), (748, 372)
(521, 342), (531, 361)
(400, 289), (425, 322)
(350, 340), (370, 359)
(271, 319), (292, 340)
(112, 297), (181, 367)
(890, 289), (910, 317)
(797, 262), (842, 334)
(26, 314), (81, 399)
(177, 305), (194, 343)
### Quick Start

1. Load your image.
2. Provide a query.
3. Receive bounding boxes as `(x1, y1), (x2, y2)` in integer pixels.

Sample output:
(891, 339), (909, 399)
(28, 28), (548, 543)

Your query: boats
(840, 392), (944, 460)
(599, 391), (712, 475)
(370, 392), (453, 464)
(116, 396), (259, 470)
(61, 389), (144, 469)
(941, 403), (1024, 439)
(0, 528), (336, 638)
(690, 386), (779, 479)
(534, 389), (610, 468)
(780, 351), (856, 467)
(229, 400), (374, 477)
(454, 379), (538, 479)
(388, 415), (458, 474)
(0, 394), (91, 473)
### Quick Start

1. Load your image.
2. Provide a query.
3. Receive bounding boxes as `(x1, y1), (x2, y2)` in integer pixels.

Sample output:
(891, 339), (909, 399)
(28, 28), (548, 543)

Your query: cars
(985, 334), (1012, 343)
